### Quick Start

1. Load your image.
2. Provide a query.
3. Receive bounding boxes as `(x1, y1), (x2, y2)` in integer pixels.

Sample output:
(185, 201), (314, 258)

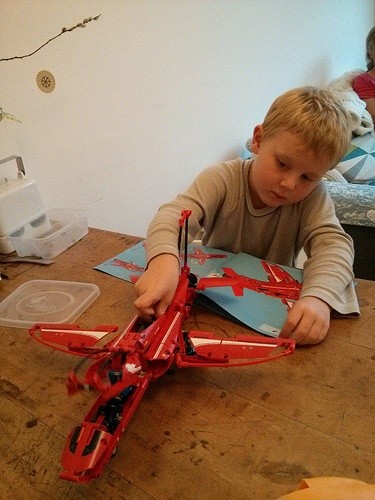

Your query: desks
(0, 220), (375, 500)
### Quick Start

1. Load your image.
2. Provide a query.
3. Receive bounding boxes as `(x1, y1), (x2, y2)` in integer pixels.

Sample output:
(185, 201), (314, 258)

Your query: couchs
(240, 119), (375, 281)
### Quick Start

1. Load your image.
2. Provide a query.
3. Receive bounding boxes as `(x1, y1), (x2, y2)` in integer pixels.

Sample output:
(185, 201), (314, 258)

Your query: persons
(350, 23), (375, 127)
(130, 83), (364, 349)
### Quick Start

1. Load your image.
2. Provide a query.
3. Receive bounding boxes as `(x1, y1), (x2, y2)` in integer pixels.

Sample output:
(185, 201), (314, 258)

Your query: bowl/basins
(6, 207), (89, 260)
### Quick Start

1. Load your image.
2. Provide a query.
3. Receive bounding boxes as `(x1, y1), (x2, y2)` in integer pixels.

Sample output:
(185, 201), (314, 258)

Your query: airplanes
(25, 205), (298, 487)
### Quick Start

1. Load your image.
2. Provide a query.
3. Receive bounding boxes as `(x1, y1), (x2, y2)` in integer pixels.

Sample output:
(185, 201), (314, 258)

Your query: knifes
(0, 256), (53, 266)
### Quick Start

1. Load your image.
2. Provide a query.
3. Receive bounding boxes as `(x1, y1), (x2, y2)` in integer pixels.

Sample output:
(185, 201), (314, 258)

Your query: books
(93, 237), (359, 340)
